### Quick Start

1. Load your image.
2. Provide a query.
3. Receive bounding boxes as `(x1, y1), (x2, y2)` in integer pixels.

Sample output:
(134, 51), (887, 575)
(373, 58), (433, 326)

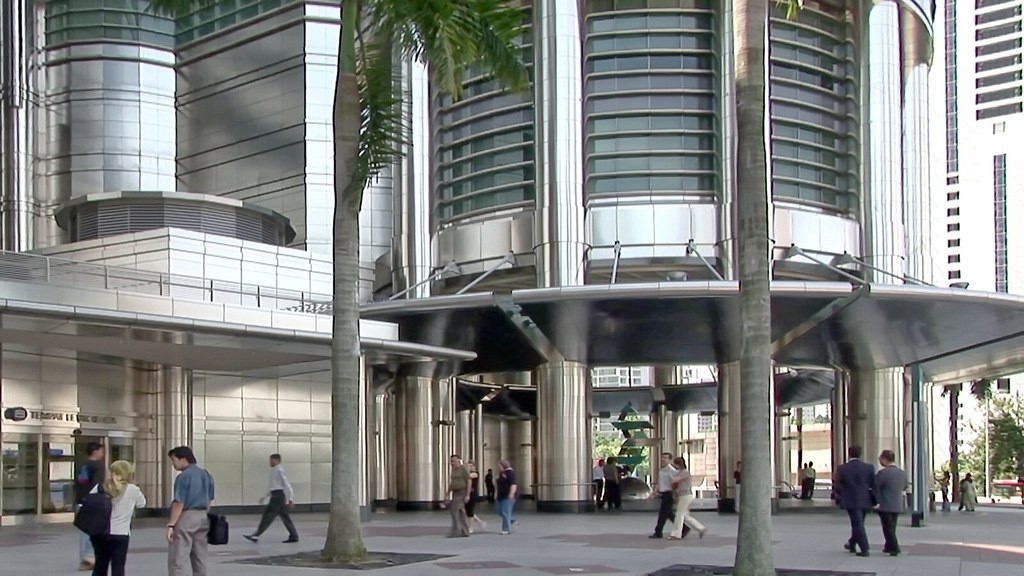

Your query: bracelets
(167, 524), (175, 529)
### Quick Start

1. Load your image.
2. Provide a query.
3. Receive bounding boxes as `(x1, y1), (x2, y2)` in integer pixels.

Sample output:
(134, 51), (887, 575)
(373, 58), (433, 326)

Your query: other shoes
(667, 536), (682, 540)
(243, 534), (258, 543)
(511, 520), (520, 526)
(699, 527), (707, 538)
(856, 550), (870, 556)
(282, 538), (299, 543)
(844, 543), (856, 552)
(882, 548), (890, 553)
(648, 533), (663, 538)
(890, 547), (901, 556)
(498, 531), (509, 535)
(682, 527), (690, 537)
(77, 556), (95, 570)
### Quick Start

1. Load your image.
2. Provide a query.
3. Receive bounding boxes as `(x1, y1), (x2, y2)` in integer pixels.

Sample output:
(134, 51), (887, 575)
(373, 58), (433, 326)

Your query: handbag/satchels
(207, 512), (230, 545)
(73, 483), (112, 538)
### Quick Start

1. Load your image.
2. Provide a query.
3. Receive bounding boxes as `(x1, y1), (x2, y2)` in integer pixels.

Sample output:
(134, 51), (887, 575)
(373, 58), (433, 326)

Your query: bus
(991, 478), (1024, 505)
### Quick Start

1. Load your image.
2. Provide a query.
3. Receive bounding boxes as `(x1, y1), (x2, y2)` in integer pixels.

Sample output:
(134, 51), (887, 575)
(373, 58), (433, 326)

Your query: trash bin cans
(718, 486), (735, 512)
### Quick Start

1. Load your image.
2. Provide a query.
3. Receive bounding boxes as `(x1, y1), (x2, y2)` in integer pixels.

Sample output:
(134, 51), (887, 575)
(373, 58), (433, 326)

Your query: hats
(85, 442), (105, 455)
(674, 458), (687, 469)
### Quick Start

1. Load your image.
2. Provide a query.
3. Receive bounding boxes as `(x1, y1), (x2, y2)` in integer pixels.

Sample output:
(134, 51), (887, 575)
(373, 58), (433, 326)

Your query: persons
(484, 468), (496, 503)
(242, 454), (299, 543)
(167, 446), (215, 576)
(495, 459), (518, 535)
(592, 457), (623, 512)
(833, 445), (880, 557)
(88, 460), (147, 576)
(934, 471), (950, 512)
(733, 461), (741, 510)
(648, 453), (707, 540)
(74, 441), (106, 571)
(876, 449), (909, 556)
(447, 454), (487, 537)
(798, 461), (816, 500)
(958, 472), (978, 512)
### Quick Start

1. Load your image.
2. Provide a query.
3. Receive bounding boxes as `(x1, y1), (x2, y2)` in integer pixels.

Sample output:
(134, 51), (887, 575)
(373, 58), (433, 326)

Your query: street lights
(985, 377), (1010, 498)
